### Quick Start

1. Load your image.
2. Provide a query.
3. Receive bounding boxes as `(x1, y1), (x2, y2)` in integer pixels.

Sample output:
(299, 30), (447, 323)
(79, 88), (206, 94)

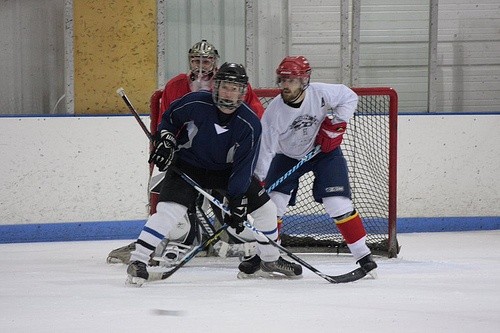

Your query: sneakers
(125, 259), (149, 287)
(259, 258), (303, 279)
(237, 255), (260, 279)
(357, 251), (378, 279)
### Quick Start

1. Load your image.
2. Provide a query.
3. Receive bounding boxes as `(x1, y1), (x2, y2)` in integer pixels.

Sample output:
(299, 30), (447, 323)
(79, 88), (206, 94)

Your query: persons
(156, 38), (263, 267)
(237, 56), (378, 280)
(125, 61), (303, 286)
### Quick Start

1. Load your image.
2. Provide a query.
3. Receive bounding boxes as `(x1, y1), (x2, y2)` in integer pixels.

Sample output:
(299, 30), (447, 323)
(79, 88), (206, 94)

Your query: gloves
(316, 116), (347, 153)
(221, 193), (248, 234)
(148, 129), (176, 171)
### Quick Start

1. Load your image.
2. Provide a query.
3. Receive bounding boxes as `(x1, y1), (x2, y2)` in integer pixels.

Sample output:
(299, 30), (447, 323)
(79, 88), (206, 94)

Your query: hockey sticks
(169, 161), (367, 284)
(116, 88), (261, 258)
(161, 144), (322, 279)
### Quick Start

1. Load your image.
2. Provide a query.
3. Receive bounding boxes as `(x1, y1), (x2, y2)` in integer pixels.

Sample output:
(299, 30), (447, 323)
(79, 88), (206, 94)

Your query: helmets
(188, 40), (218, 79)
(212, 61), (249, 109)
(275, 54), (312, 89)
